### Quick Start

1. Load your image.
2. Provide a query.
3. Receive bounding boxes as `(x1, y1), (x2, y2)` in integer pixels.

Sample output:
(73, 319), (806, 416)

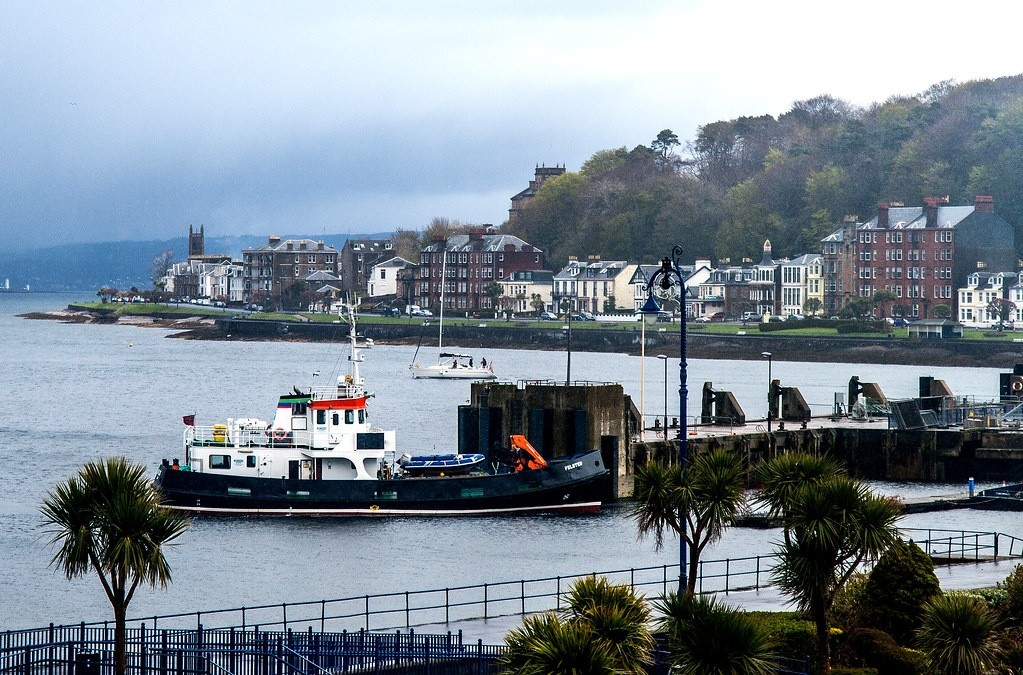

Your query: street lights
(657, 354), (668, 441)
(761, 351), (772, 431)
(632, 245), (713, 600)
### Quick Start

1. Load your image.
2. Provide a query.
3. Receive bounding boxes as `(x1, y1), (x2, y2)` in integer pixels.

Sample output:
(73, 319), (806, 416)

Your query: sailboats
(408, 250), (499, 379)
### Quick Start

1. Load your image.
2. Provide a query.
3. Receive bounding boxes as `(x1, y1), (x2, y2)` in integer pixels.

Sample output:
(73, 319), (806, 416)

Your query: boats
(149, 285), (610, 520)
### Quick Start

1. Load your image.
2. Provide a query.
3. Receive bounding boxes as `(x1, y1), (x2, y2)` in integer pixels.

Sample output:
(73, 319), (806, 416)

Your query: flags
(183, 415), (195, 426)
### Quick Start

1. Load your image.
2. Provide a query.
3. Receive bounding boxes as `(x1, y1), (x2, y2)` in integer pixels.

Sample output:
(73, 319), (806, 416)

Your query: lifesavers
(265, 424), (272, 436)
(273, 427), (287, 440)
(1012, 381), (1023, 391)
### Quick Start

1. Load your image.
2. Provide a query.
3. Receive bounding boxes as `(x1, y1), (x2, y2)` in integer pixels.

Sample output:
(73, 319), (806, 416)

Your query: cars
(868, 316), (911, 327)
(696, 312), (725, 323)
(243, 304), (257, 310)
(770, 315), (787, 323)
(580, 312), (596, 322)
(417, 310), (433, 317)
(991, 319), (1015, 330)
(541, 312), (558, 320)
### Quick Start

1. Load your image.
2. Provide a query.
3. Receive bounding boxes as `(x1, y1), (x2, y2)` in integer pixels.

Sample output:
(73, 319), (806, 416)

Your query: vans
(405, 305), (420, 314)
(741, 312), (762, 321)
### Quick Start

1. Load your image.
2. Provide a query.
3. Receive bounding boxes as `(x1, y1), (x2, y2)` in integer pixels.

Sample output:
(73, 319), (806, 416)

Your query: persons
(515, 454), (544, 473)
